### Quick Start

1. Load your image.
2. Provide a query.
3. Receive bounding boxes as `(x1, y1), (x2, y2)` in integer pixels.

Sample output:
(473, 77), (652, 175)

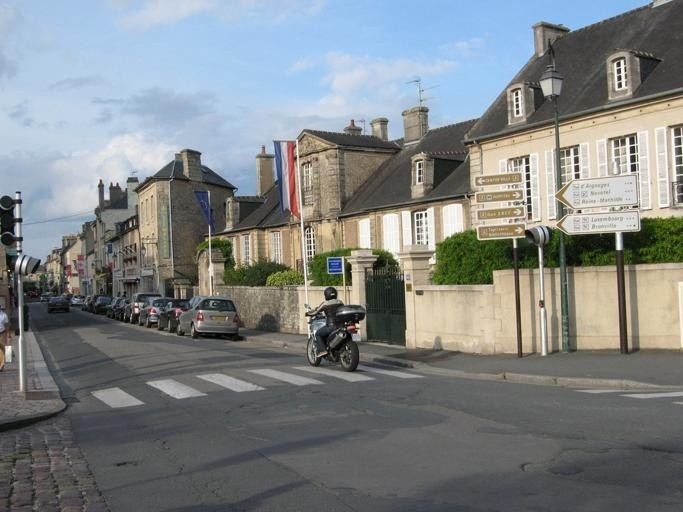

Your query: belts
(0, 329), (6, 333)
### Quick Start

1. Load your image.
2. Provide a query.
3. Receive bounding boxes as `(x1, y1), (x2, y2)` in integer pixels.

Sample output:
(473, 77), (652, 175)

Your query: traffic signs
(553, 172), (641, 237)
(474, 172), (527, 240)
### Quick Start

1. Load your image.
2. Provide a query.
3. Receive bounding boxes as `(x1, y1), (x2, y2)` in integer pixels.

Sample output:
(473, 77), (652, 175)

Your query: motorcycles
(303, 304), (365, 371)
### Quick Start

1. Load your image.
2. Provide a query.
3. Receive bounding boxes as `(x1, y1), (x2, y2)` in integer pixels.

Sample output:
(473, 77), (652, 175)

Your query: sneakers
(317, 351), (328, 357)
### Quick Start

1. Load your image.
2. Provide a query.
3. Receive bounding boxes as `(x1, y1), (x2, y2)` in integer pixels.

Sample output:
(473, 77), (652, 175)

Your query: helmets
(324, 286), (338, 299)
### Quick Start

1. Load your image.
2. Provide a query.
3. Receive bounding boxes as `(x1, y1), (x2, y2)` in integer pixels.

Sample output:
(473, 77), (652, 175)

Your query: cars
(26, 289), (240, 339)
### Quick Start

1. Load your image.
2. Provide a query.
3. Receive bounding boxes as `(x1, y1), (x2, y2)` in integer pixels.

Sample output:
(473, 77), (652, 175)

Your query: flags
(274, 140), (301, 220)
(194, 191), (217, 231)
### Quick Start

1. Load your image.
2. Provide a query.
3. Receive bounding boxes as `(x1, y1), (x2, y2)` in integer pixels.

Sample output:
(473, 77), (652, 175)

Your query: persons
(117, 292), (120, 297)
(305, 286), (344, 359)
(0, 305), (11, 372)
(122, 291), (127, 297)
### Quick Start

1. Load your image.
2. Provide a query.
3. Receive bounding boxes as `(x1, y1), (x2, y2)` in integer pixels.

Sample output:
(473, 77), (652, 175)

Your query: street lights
(540, 41), (572, 354)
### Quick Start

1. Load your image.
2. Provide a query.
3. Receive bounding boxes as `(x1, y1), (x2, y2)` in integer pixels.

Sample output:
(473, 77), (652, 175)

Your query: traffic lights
(6, 254), (41, 276)
(0, 195), (14, 245)
(525, 224), (554, 247)
